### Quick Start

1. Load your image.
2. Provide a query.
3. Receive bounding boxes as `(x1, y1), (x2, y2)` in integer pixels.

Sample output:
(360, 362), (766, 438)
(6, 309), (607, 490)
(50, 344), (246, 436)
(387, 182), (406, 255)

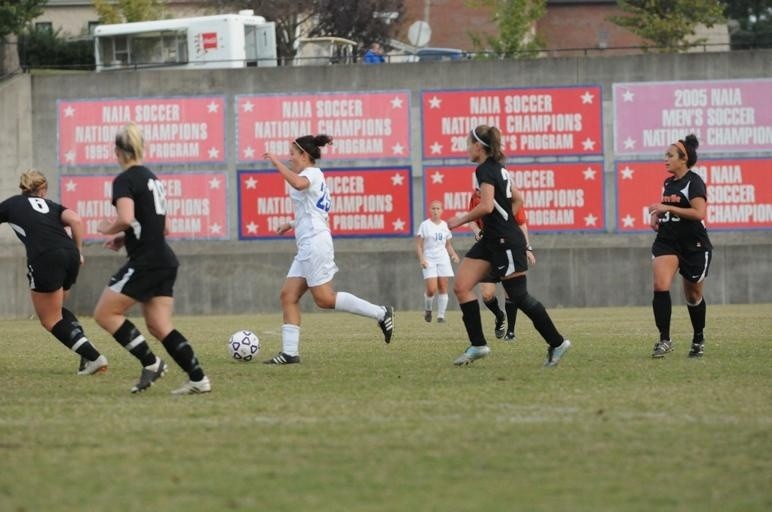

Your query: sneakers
(424, 310), (432, 322)
(544, 337), (571, 367)
(170, 376), (211, 395)
(651, 337), (674, 358)
(454, 346), (491, 365)
(688, 338), (705, 359)
(494, 311), (506, 339)
(262, 352), (299, 364)
(376, 305), (395, 345)
(131, 356), (168, 394)
(77, 355), (108, 375)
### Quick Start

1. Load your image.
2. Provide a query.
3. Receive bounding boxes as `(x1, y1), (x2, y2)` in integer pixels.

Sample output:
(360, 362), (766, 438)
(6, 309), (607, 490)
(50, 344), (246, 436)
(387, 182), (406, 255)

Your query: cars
(415, 48), (472, 63)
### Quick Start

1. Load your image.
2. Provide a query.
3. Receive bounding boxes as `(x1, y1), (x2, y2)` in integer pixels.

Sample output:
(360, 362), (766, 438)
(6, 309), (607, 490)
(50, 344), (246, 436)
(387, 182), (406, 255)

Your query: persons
(87, 118), (211, 395)
(648, 133), (714, 359)
(446, 125), (573, 368)
(415, 198), (462, 323)
(465, 151), (536, 341)
(0, 167), (109, 374)
(363, 41), (387, 64)
(263, 134), (397, 365)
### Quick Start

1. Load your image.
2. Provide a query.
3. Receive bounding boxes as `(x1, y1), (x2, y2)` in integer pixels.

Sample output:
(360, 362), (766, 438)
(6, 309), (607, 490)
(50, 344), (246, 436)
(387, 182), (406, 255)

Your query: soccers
(228, 330), (259, 361)
